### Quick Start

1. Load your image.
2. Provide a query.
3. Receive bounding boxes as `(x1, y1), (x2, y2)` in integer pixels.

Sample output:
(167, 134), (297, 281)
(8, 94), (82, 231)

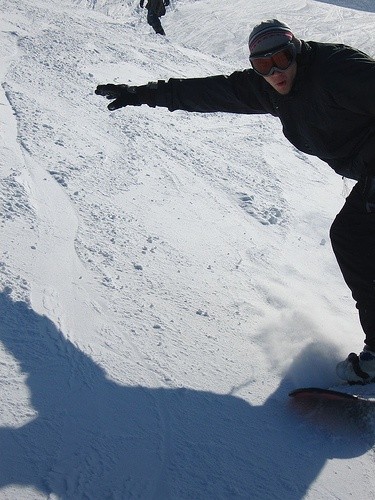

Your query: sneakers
(337, 339), (375, 386)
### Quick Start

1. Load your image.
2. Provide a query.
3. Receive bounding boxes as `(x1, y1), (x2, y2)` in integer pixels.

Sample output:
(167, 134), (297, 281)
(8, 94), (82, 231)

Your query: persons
(93, 19), (375, 383)
(140, 0), (170, 35)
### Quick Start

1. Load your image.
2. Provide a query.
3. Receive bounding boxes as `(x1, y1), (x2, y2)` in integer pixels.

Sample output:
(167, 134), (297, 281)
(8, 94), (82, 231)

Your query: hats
(248, 19), (293, 55)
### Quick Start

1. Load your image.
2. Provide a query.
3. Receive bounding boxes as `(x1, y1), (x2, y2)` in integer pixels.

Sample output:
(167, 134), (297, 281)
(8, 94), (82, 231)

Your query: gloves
(139, 0), (144, 9)
(95, 82), (157, 112)
(163, 0), (169, 6)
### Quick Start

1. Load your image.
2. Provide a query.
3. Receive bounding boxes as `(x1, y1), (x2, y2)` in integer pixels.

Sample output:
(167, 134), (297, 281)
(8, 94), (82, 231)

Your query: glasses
(249, 41), (296, 76)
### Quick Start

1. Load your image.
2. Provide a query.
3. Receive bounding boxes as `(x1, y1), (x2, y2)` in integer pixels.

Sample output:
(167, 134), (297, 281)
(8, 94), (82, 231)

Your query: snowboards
(288, 382), (375, 405)
